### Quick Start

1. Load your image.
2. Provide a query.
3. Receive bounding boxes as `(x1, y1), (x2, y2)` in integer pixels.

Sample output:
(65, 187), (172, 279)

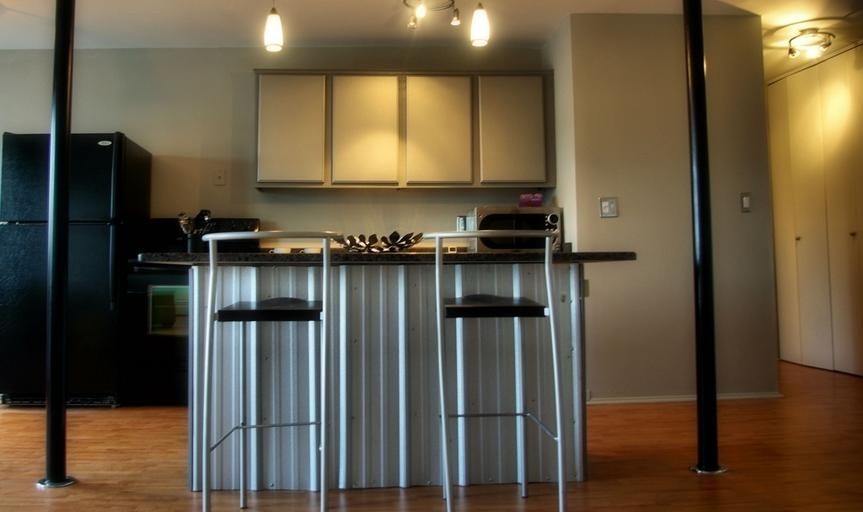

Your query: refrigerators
(1, 131), (153, 408)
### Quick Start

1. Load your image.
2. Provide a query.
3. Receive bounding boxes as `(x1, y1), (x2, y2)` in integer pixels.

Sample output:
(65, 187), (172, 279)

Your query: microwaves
(475, 207), (562, 254)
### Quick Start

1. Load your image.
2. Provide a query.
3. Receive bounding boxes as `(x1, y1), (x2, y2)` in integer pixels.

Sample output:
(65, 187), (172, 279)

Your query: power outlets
(599, 197), (619, 218)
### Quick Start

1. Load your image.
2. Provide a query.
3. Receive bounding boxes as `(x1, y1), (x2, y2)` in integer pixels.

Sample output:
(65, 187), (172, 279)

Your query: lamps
(403, 0), (461, 34)
(789, 28), (836, 59)
(263, 0), (283, 52)
(471, 0), (490, 47)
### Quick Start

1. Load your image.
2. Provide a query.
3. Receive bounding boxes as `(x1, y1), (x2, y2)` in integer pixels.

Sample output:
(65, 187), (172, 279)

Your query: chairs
(420, 229), (564, 512)
(202, 232), (347, 512)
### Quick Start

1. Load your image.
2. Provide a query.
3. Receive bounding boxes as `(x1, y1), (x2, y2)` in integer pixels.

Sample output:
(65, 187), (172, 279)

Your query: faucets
(186, 209), (211, 254)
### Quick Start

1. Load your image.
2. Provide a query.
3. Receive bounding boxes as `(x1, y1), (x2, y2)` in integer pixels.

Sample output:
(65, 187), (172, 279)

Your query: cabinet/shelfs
(254, 73), (400, 185)
(400, 72), (554, 185)
(769, 63), (862, 378)
